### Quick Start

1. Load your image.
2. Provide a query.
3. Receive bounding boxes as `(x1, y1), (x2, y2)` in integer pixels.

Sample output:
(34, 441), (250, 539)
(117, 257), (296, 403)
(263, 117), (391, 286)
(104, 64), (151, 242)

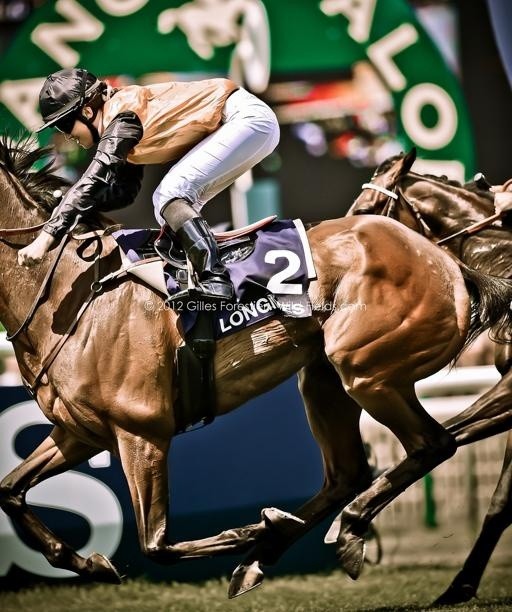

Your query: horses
(0, 125), (511, 600)
(344, 147), (512, 608)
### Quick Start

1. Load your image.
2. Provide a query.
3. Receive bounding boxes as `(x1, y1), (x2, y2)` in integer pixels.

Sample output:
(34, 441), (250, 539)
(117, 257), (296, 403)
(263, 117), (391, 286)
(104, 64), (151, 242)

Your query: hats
(35, 69), (102, 133)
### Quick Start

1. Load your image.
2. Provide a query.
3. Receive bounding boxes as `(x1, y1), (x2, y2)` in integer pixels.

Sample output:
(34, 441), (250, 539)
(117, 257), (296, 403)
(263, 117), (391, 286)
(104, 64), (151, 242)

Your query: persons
(490, 179), (512, 214)
(15, 66), (284, 315)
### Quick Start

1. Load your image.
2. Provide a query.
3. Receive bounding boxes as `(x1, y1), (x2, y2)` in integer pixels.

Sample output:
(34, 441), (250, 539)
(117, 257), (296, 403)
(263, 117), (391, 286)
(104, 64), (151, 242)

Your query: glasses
(54, 69), (87, 134)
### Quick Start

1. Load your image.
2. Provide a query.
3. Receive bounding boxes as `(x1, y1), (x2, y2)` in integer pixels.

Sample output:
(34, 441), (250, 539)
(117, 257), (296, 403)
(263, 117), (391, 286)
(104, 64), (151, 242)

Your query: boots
(165, 216), (236, 308)
